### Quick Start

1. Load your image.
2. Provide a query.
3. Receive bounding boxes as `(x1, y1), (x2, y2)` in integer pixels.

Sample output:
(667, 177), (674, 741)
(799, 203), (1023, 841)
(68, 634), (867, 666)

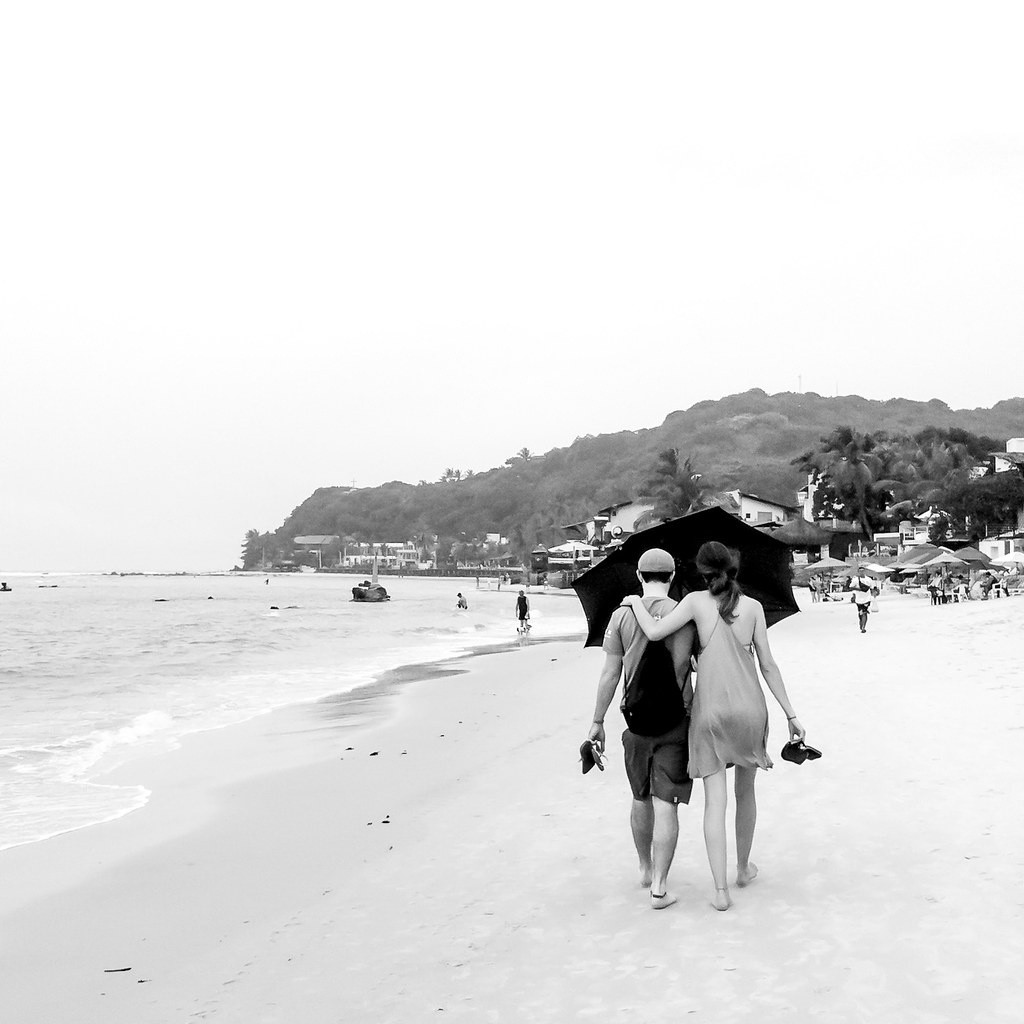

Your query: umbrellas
(888, 542), (1024, 587)
(804, 557), (852, 582)
(571, 504), (801, 658)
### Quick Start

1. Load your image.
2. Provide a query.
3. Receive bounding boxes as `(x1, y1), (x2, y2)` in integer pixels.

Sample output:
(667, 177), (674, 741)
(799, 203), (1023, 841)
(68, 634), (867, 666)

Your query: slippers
(781, 741), (823, 764)
(578, 738), (606, 775)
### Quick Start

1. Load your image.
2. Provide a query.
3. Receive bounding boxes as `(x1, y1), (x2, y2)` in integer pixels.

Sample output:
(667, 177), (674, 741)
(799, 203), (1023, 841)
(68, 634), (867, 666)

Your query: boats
(0, 582), (12, 591)
(352, 580), (390, 603)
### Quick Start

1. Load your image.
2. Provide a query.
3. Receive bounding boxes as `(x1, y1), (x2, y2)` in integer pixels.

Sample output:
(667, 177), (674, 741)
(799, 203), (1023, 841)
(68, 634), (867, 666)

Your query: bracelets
(787, 717), (796, 720)
(594, 720), (604, 724)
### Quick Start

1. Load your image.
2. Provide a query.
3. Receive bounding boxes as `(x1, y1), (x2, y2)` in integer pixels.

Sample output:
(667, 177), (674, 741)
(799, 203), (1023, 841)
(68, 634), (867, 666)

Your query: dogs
(517, 624), (532, 637)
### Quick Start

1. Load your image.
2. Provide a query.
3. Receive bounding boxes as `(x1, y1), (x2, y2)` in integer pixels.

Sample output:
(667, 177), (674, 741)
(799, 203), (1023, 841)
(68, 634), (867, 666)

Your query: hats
(638, 548), (676, 574)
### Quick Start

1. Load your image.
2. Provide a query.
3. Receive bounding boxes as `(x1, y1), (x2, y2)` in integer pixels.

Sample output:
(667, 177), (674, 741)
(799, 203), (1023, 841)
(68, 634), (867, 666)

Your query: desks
(824, 582), (841, 594)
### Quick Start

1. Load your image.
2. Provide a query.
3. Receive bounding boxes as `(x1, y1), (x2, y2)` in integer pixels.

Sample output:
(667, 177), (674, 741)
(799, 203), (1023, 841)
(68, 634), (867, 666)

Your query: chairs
(988, 578), (1005, 599)
(951, 584), (968, 604)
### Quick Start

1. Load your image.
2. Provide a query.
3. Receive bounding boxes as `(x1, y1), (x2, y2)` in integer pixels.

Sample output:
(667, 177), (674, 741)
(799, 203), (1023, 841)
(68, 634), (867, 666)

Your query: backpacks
(620, 605), (696, 737)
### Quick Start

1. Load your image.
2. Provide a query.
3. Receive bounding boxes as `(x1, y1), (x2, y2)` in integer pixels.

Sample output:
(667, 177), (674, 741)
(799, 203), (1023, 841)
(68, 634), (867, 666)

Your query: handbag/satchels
(858, 577), (872, 593)
(871, 595), (879, 612)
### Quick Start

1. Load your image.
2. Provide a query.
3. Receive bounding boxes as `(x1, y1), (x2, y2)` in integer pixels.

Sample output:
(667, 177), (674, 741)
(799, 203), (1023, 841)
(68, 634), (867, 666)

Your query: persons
(810, 574), (821, 603)
(516, 590), (530, 627)
(620, 542), (806, 910)
(457, 593), (468, 609)
(927, 573), (999, 605)
(587, 548), (698, 909)
(849, 567), (880, 633)
(843, 575), (852, 591)
